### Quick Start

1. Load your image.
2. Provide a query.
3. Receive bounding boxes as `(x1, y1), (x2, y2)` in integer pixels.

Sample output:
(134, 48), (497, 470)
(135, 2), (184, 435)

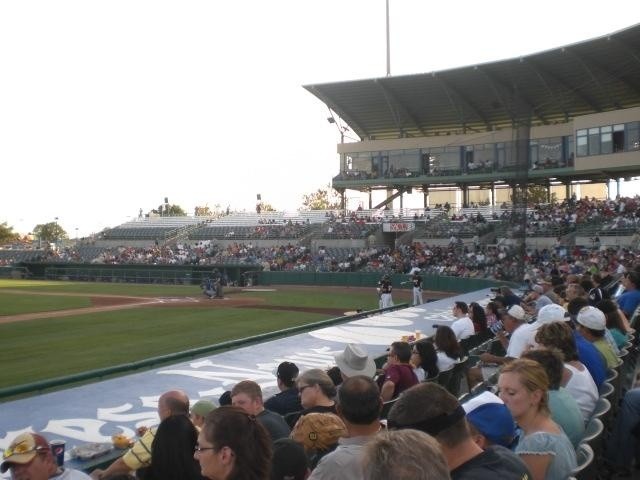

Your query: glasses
(2, 440), (43, 460)
(194, 446), (218, 454)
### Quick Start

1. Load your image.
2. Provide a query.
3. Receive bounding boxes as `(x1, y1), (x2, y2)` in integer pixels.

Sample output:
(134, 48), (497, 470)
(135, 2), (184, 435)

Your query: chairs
(100, 207), (640, 240)
(424, 280), (640, 480)
(63, 342), (422, 478)
(359, 246), (639, 278)
(2, 246), (385, 271)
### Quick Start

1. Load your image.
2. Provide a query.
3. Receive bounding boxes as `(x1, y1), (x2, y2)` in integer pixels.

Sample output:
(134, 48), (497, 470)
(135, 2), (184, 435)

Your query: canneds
(490, 320), (504, 335)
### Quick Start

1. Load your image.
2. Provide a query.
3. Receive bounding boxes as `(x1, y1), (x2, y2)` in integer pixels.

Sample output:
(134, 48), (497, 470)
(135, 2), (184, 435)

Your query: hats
(588, 288), (610, 305)
(0, 433), (49, 473)
(573, 305), (607, 330)
(272, 361), (299, 388)
(529, 284), (544, 294)
(498, 304), (525, 321)
(188, 399), (217, 418)
(462, 389), (516, 445)
(528, 303), (571, 331)
(335, 343), (377, 379)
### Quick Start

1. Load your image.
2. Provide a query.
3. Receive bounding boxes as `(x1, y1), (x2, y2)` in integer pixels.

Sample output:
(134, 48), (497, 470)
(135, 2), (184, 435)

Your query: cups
(113, 434), (131, 450)
(491, 319), (504, 336)
(50, 439), (66, 466)
(403, 330), (421, 342)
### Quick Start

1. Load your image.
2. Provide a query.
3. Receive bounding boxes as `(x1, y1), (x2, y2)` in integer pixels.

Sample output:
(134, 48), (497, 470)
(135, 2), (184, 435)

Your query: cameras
(490, 287), (502, 294)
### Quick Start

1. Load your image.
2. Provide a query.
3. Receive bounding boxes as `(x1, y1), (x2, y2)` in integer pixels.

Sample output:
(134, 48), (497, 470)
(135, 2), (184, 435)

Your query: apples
(114, 435), (128, 450)
